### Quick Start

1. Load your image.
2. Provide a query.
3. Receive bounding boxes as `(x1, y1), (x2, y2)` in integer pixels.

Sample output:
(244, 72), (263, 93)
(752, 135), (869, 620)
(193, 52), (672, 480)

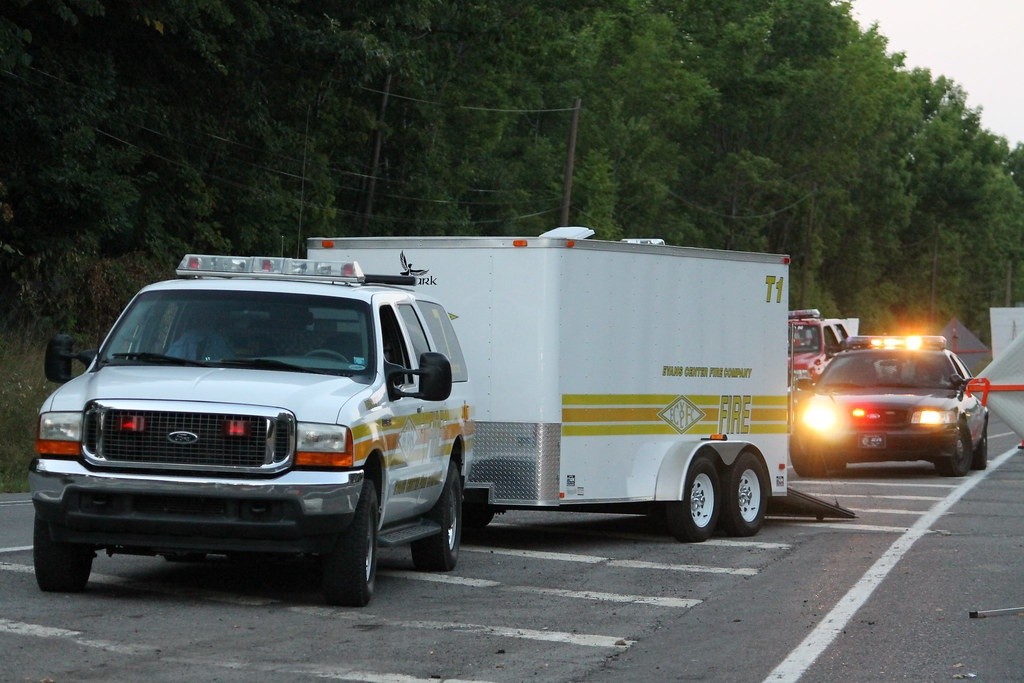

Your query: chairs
(166, 328), (231, 361)
(318, 332), (362, 358)
(838, 361), (870, 384)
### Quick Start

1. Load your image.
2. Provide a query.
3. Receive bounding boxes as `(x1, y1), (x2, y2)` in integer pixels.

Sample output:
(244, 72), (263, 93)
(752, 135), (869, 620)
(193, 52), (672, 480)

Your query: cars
(787, 306), (853, 390)
(788, 335), (992, 478)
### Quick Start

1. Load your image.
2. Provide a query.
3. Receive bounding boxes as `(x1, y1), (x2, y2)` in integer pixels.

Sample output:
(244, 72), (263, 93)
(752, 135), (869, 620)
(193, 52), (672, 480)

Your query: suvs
(28, 252), (474, 611)
